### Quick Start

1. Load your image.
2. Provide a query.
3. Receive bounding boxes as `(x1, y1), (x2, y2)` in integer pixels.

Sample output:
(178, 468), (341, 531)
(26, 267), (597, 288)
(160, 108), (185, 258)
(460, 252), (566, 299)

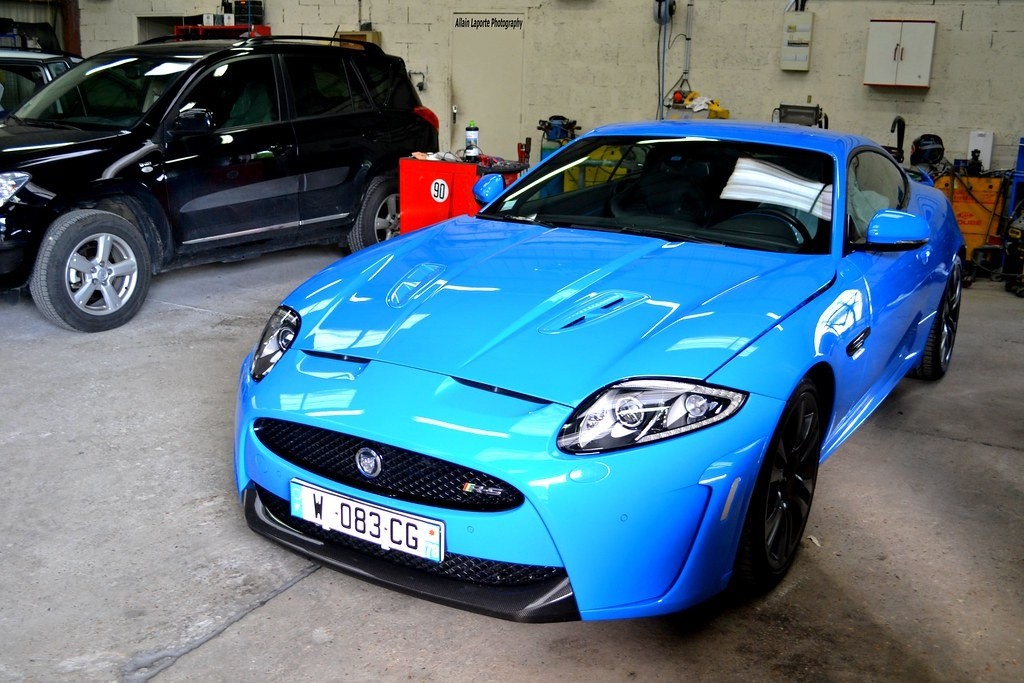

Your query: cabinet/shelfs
(863, 20), (938, 90)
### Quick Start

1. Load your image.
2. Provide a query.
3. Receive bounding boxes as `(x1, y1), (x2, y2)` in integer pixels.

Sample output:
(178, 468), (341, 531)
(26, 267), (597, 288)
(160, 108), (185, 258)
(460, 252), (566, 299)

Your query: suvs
(0, 23), (440, 332)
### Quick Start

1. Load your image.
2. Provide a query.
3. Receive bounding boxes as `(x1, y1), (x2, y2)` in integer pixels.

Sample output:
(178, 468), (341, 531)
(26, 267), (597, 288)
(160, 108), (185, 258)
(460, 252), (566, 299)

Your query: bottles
(465, 121), (479, 161)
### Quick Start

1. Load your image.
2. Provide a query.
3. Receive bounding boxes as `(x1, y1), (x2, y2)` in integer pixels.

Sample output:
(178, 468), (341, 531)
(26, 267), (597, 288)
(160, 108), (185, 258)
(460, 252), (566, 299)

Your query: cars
(0, 47), (144, 122)
(229, 119), (966, 624)
